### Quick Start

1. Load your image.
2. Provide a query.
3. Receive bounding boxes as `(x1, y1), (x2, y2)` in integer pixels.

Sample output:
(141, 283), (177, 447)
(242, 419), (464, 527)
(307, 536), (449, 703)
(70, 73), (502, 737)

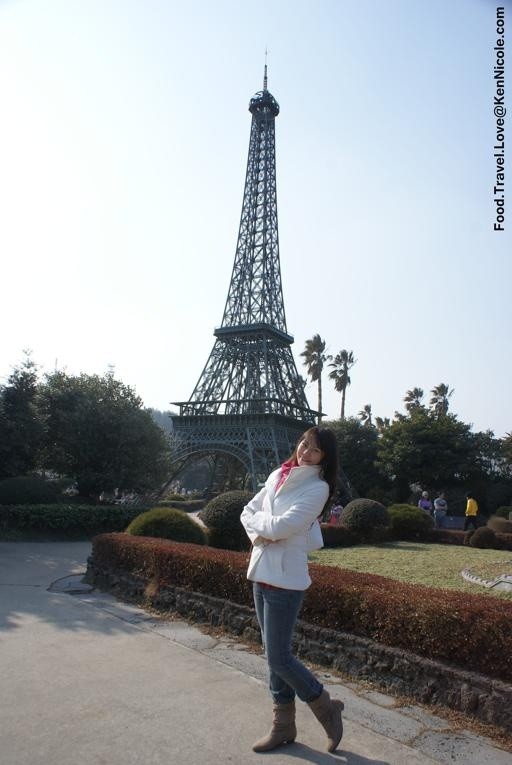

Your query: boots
(252, 688), (344, 753)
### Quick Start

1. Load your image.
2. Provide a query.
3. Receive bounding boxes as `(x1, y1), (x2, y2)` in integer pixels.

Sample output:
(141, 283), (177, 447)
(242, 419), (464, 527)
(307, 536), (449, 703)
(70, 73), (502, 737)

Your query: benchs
(442, 515), (474, 530)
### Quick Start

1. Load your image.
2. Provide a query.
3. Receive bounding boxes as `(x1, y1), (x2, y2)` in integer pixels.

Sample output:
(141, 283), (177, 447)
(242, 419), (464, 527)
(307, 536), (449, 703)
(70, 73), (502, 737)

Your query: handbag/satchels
(306, 519), (324, 553)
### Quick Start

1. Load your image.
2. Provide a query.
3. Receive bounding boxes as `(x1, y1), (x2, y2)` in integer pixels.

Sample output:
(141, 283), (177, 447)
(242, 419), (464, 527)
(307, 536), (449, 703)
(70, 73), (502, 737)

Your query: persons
(240, 427), (344, 752)
(463, 491), (479, 531)
(330, 500), (343, 520)
(434, 490), (449, 527)
(417, 490), (432, 511)
